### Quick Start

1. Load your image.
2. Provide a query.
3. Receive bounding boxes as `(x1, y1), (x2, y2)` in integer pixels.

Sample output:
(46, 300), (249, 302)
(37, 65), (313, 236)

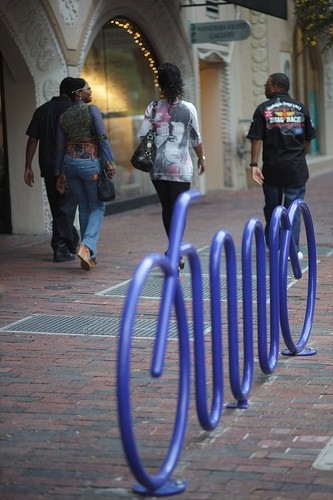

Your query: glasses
(72, 86), (91, 94)
(264, 83), (274, 88)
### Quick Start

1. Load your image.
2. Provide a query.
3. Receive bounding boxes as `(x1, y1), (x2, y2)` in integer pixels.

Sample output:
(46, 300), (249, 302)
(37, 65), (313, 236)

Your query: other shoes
(88, 258), (96, 268)
(78, 245), (91, 270)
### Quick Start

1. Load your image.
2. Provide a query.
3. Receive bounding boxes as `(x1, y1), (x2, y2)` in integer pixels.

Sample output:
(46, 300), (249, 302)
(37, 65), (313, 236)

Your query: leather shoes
(71, 239), (80, 254)
(53, 243), (76, 262)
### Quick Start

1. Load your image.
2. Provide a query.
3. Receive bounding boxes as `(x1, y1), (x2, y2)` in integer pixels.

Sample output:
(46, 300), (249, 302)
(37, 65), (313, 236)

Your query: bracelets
(197, 156), (205, 160)
(250, 163), (258, 166)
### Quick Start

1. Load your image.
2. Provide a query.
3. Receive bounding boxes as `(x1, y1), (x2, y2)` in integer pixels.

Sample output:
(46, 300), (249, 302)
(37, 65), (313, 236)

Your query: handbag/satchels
(97, 167), (115, 202)
(130, 130), (156, 172)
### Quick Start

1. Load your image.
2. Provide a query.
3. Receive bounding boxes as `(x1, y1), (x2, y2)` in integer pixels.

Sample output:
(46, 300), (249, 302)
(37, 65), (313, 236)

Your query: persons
(24, 77), (79, 262)
(55, 78), (116, 271)
(247, 73), (316, 260)
(139, 62), (206, 271)
